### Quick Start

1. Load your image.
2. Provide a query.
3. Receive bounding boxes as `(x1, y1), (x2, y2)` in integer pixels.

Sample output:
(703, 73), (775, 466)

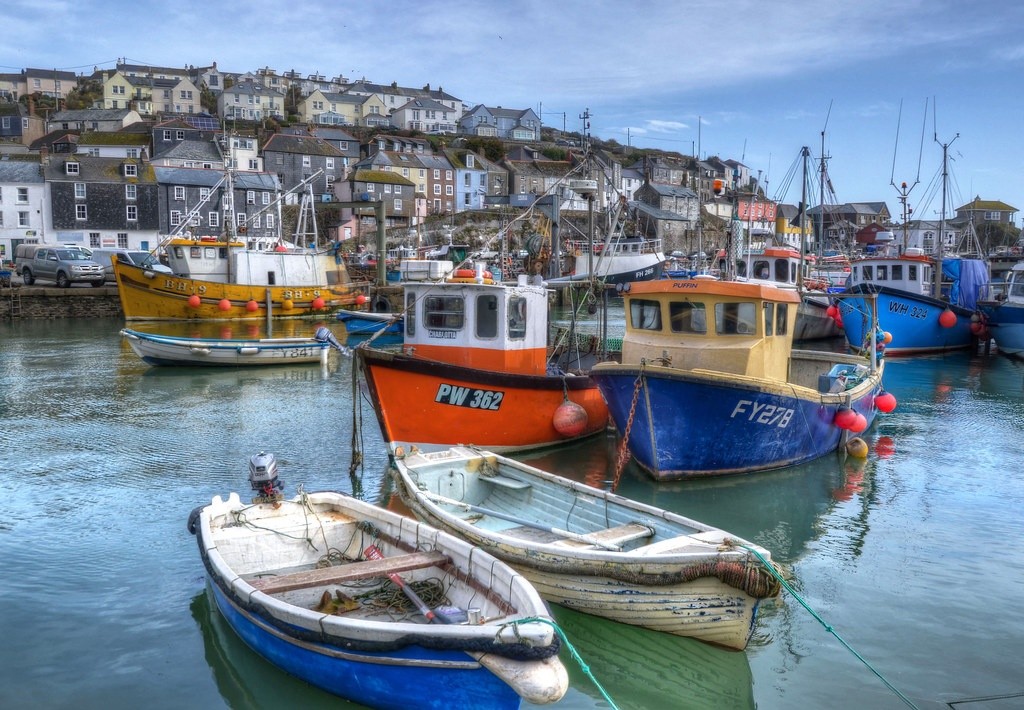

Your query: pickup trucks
(16, 243), (105, 289)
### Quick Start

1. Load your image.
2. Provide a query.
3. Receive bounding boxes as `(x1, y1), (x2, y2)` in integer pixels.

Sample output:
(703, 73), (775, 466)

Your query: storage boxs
(829, 363), (856, 375)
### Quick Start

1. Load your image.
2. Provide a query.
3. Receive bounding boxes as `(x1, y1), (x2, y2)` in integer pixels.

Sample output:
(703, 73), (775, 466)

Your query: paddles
(421, 490), (623, 552)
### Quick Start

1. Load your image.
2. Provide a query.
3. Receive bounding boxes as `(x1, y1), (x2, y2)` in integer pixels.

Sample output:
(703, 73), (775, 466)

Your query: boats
(540, 135), (1024, 359)
(118, 328), (332, 364)
(588, 146), (885, 482)
(354, 151), (609, 451)
(186, 451), (569, 710)
(386, 443), (785, 651)
(109, 166), (371, 320)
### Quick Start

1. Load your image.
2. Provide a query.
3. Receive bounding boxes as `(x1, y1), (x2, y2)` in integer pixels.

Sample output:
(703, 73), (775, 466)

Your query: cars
(687, 250), (707, 260)
(665, 249), (686, 259)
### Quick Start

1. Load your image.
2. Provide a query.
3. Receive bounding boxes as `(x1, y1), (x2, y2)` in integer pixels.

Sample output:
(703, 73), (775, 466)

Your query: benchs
(247, 550), (452, 595)
(543, 521), (655, 552)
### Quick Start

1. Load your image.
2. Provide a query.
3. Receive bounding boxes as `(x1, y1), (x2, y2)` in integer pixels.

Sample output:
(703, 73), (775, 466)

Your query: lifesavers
(201, 237), (216, 242)
(1012, 246), (1020, 255)
(452, 269), (493, 279)
(371, 296), (392, 313)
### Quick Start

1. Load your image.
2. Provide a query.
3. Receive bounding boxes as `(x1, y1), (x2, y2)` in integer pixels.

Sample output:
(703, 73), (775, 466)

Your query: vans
(713, 249), (726, 258)
(90, 248), (174, 286)
